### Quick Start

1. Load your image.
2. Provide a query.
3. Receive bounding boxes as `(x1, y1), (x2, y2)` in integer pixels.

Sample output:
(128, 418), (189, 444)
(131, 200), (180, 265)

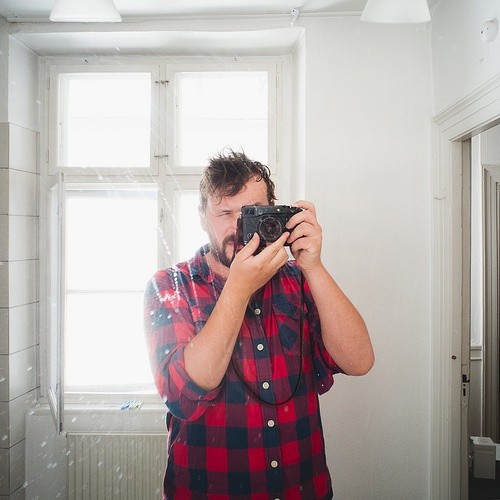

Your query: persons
(144, 146), (374, 500)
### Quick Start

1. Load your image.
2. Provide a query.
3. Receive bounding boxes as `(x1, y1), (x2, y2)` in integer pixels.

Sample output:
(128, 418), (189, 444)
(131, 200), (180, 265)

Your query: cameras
(240, 205), (304, 247)
